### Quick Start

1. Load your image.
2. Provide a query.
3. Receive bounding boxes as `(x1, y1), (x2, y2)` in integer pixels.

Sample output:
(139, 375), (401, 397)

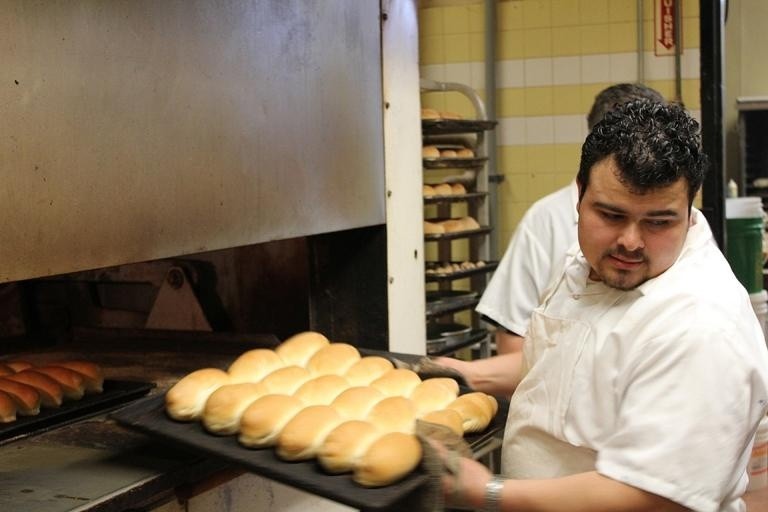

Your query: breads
(161, 328), (501, 490)
(1, 361), (104, 425)
(421, 108), (487, 279)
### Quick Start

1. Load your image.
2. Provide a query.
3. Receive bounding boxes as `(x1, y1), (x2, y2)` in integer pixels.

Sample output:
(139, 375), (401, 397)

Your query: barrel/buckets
(750, 291), (767, 339)
(727, 218), (764, 291)
(747, 418), (767, 489)
(728, 194), (766, 218)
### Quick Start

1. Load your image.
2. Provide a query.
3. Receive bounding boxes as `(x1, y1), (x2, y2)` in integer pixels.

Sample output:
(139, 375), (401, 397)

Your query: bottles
(728, 180), (739, 197)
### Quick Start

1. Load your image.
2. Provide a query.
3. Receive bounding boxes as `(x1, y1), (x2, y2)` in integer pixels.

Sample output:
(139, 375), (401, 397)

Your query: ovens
(2, 2), (428, 512)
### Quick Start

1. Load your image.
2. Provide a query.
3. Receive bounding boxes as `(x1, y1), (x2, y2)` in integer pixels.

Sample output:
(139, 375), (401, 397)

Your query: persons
(475, 83), (664, 405)
(430, 96), (768, 512)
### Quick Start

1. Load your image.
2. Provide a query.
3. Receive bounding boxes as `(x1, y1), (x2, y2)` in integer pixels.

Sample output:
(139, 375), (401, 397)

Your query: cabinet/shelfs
(421, 77), (497, 357)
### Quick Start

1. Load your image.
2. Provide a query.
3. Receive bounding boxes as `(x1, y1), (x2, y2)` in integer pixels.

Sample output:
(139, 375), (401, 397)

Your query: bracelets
(481, 472), (510, 512)
